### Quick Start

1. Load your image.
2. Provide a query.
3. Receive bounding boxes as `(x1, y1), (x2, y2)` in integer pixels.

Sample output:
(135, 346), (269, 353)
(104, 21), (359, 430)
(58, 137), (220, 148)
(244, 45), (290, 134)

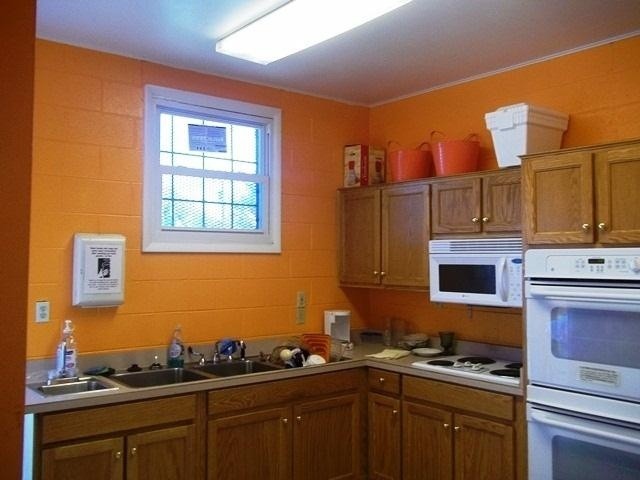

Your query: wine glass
(439, 331), (455, 356)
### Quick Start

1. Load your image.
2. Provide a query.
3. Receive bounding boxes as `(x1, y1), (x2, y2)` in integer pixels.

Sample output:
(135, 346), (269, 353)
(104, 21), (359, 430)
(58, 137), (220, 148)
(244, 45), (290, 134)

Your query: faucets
(212, 339), (246, 364)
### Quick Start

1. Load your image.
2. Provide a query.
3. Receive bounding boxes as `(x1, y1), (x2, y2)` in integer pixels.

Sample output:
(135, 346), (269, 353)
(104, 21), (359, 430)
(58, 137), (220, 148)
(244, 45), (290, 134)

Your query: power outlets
(36, 301), (49, 323)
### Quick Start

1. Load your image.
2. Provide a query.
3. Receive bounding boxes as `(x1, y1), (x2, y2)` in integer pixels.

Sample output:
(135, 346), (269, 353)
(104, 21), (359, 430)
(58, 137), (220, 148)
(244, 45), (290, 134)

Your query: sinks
(189, 360), (278, 379)
(253, 352), (352, 370)
(105, 367), (208, 388)
(28, 376), (119, 399)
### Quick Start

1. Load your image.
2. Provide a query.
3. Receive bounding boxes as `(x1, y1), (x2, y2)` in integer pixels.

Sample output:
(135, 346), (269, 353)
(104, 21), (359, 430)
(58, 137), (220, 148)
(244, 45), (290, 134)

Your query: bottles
(347, 161), (356, 185)
(383, 319), (393, 346)
(375, 158), (382, 177)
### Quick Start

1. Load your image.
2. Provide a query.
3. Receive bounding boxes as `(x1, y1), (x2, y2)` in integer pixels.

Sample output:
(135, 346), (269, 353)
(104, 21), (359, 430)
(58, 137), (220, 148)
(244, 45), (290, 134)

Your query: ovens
(523, 247), (640, 480)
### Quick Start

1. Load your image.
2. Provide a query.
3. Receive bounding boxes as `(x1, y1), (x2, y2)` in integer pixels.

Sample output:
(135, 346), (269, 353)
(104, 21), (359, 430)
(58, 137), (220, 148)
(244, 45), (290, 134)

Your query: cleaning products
(56, 320), (78, 378)
(168, 327), (184, 368)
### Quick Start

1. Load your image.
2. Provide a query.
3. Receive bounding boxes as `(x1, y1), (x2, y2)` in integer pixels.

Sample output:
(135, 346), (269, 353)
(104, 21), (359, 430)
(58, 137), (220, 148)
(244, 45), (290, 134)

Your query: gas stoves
(411, 353), (523, 389)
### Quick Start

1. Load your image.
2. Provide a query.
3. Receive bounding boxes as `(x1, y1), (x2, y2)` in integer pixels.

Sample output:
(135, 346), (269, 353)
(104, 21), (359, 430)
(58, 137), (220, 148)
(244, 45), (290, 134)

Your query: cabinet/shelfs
(365, 367), (400, 480)
(206, 366), (367, 480)
(336, 179), (430, 288)
(35, 390), (208, 480)
(431, 173), (521, 238)
(516, 139), (640, 247)
(401, 375), (525, 480)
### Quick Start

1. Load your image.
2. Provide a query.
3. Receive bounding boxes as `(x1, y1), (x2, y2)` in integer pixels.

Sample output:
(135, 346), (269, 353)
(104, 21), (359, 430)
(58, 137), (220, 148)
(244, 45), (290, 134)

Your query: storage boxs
(485, 103), (570, 168)
(343, 143), (386, 189)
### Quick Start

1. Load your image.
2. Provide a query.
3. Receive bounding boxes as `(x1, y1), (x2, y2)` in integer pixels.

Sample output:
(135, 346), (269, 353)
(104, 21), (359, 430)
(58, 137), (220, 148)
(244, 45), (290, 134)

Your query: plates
(302, 334), (330, 363)
(412, 348), (442, 357)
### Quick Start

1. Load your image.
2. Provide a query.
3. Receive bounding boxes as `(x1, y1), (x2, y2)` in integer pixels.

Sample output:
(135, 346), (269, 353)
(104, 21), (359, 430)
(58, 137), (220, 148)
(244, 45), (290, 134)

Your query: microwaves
(429, 238), (524, 307)
